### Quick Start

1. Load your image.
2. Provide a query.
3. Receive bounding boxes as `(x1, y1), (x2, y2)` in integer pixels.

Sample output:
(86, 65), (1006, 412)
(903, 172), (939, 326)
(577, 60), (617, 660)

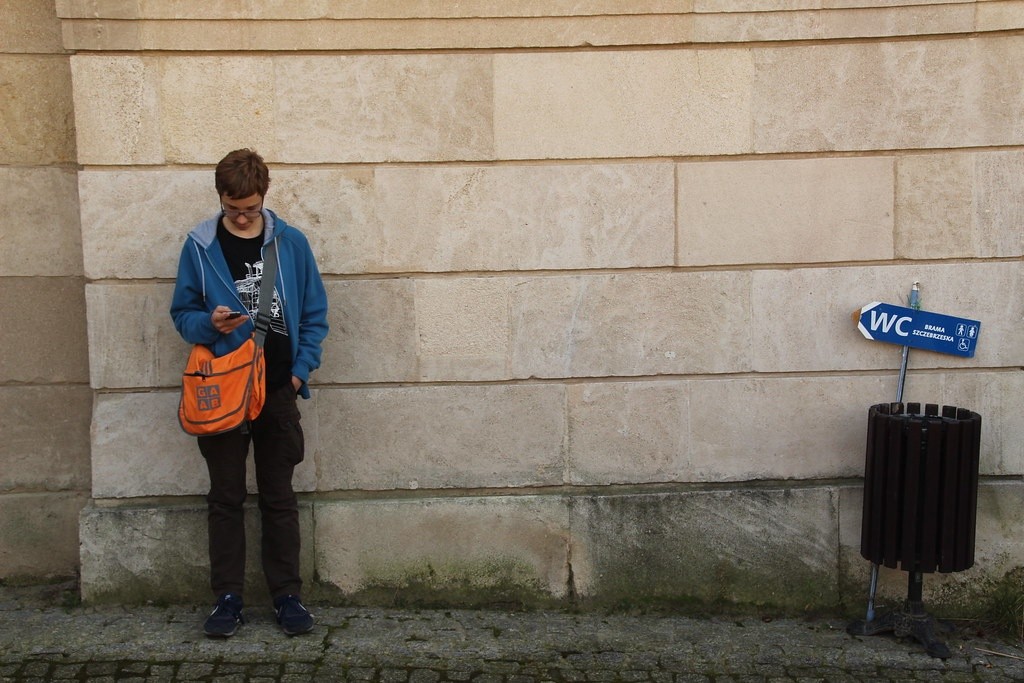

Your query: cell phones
(223, 311), (240, 321)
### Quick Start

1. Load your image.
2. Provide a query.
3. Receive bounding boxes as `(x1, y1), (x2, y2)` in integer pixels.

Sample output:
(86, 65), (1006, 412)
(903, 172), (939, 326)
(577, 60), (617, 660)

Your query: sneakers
(203, 592), (247, 637)
(273, 594), (314, 636)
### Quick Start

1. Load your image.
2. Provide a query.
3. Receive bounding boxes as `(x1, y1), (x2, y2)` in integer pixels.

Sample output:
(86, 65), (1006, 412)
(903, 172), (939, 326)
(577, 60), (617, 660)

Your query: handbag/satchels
(177, 331), (266, 437)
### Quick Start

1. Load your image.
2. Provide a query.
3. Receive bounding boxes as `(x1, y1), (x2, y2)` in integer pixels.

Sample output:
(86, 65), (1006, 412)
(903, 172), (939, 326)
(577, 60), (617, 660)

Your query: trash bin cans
(861, 398), (981, 637)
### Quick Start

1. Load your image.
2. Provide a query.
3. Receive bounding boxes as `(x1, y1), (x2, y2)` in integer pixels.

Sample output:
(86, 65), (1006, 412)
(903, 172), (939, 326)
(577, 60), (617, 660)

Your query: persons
(170, 148), (329, 636)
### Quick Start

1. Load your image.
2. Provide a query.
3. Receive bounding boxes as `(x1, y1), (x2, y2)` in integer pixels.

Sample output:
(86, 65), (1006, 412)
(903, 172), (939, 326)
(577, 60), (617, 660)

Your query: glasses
(220, 194), (264, 218)
